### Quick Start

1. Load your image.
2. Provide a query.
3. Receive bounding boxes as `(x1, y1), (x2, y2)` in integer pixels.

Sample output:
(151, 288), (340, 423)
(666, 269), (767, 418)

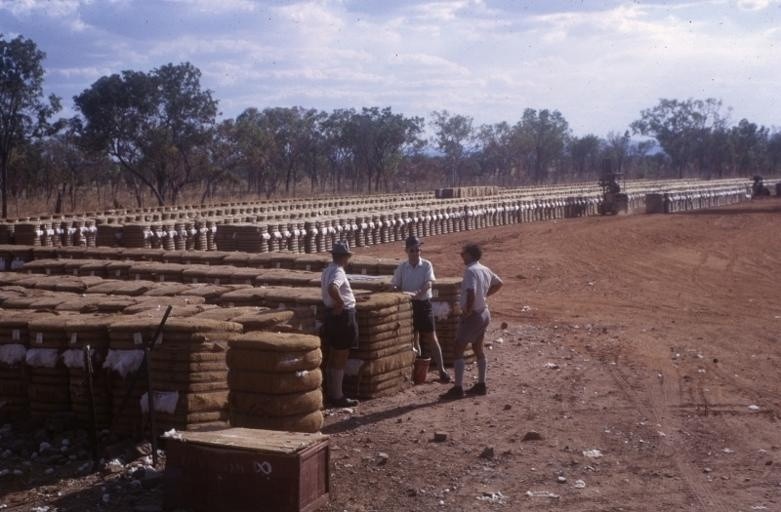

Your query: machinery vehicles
(597, 170), (630, 216)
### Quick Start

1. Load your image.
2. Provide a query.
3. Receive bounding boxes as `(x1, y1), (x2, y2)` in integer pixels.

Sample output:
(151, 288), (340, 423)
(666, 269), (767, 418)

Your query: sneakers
(325, 391), (360, 408)
(437, 370), (451, 384)
(464, 381), (488, 396)
(437, 385), (465, 400)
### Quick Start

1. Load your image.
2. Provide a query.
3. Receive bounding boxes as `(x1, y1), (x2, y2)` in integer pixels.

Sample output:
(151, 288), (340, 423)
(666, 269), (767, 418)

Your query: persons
(391, 236), (453, 382)
(321, 240), (360, 407)
(438, 242), (503, 399)
(604, 177), (620, 200)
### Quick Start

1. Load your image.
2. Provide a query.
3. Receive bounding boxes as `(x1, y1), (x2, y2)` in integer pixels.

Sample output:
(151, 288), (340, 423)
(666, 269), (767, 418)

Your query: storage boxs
(159, 427), (333, 511)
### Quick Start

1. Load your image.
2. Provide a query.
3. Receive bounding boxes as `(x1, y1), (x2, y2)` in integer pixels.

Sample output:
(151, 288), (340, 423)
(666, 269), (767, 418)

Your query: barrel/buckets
(414, 356), (432, 384)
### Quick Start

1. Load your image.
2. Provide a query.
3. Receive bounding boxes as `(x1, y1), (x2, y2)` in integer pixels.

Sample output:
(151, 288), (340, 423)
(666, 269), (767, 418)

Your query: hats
(403, 234), (426, 253)
(326, 236), (354, 258)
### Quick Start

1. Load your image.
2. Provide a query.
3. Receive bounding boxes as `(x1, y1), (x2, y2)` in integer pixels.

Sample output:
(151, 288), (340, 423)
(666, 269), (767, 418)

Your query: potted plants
(413, 342), (432, 383)
